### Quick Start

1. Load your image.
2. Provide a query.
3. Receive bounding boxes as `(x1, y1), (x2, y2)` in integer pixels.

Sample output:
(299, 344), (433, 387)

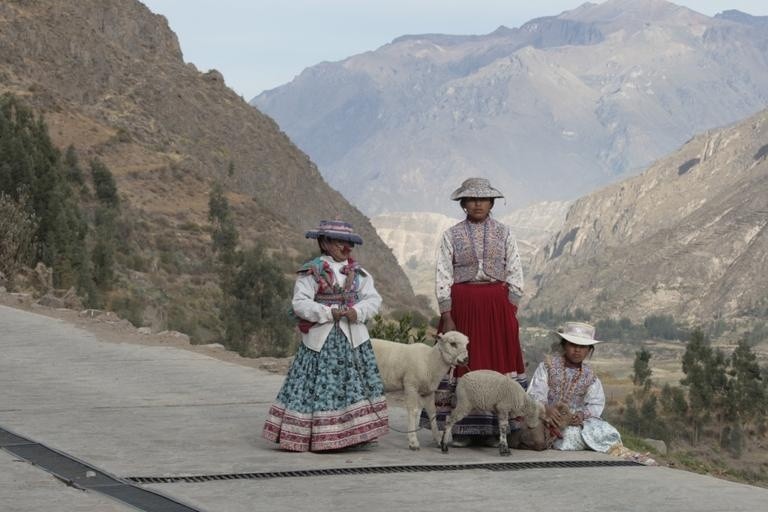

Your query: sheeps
(505, 401), (579, 452)
(368, 331), (471, 451)
(437, 369), (546, 457)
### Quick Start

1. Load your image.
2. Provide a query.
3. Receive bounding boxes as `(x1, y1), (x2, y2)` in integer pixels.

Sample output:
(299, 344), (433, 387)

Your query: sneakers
(478, 436), (501, 448)
(452, 435), (476, 447)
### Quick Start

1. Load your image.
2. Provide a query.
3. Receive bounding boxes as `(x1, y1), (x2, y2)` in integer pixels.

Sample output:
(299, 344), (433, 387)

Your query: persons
(259, 218), (390, 456)
(523, 322), (655, 465)
(416, 176), (528, 451)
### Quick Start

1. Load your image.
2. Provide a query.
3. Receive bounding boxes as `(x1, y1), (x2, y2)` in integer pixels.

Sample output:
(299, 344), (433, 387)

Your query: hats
(450, 177), (504, 201)
(305, 219), (363, 245)
(552, 321), (606, 347)
